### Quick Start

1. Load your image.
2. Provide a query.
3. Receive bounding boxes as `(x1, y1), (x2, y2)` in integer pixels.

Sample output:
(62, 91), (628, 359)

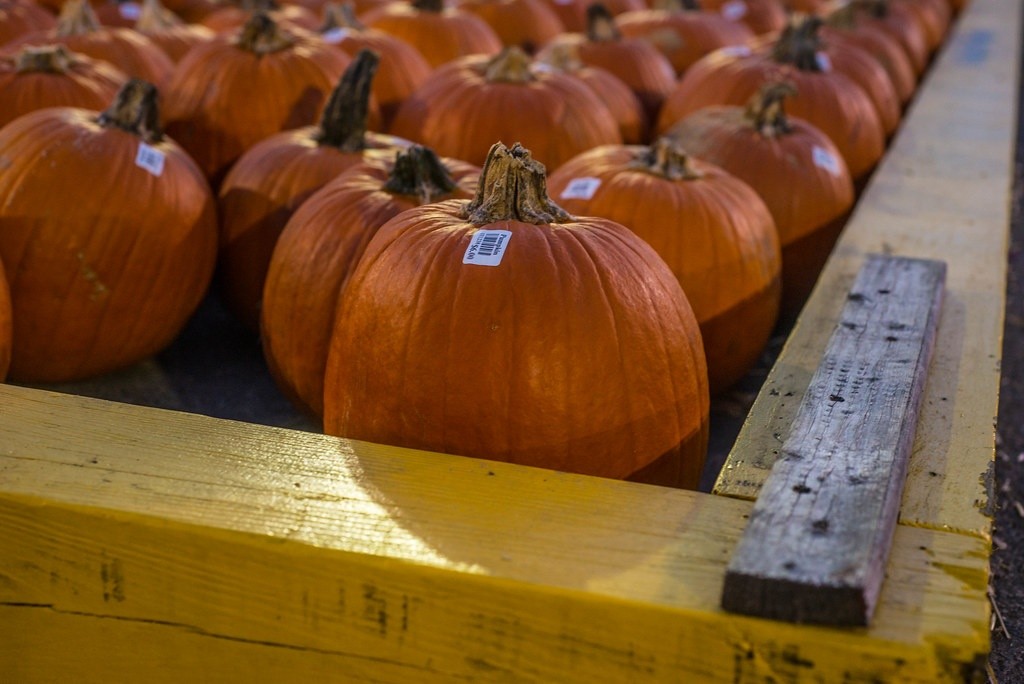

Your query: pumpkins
(0, 0), (966, 493)
